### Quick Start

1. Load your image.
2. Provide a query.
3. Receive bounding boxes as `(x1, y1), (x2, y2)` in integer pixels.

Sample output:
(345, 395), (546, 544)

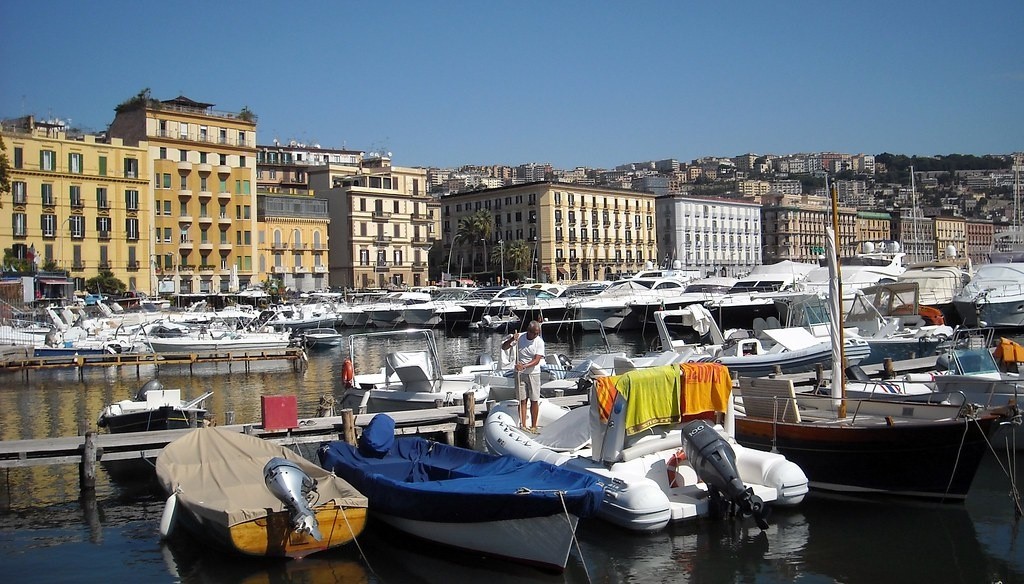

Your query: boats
(95, 380), (215, 434)
(0, 241), (1023, 412)
(814, 325), (1023, 453)
(155, 427), (369, 563)
(315, 413), (609, 569)
(481, 363), (810, 534)
(731, 226), (1023, 506)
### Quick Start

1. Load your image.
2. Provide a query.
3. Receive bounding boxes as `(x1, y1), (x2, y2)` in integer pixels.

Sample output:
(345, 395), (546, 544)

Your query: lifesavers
(666, 449), (702, 488)
(342, 359), (353, 387)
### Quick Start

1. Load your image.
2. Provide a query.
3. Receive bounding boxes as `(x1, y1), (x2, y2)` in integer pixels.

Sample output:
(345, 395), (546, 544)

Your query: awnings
(557, 267), (568, 275)
(41, 278), (76, 286)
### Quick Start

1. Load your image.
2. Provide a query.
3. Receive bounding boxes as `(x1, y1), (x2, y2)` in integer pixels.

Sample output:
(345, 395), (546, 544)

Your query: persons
(503, 321), (545, 436)
